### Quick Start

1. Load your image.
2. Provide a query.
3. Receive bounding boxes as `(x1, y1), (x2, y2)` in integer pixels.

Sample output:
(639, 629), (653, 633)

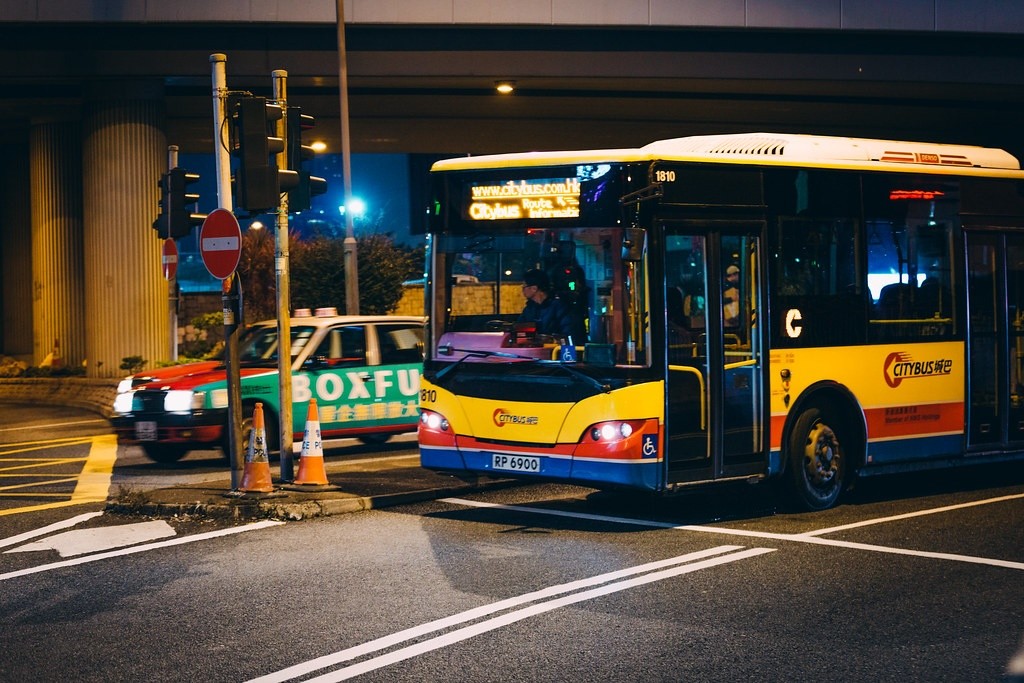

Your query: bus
(418, 133), (1024, 511)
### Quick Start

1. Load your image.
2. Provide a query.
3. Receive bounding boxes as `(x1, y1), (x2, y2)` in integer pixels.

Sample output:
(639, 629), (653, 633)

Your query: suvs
(109, 307), (427, 469)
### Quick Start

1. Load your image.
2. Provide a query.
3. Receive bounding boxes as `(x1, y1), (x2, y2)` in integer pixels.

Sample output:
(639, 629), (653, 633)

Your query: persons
(517, 269), (580, 340)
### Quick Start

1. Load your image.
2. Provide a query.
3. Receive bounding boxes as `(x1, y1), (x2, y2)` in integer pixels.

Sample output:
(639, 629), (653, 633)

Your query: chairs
(667, 285), (693, 401)
(868, 276), (952, 319)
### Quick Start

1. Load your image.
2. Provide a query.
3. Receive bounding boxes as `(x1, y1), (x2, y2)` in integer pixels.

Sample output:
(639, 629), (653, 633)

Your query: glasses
(520, 284), (534, 291)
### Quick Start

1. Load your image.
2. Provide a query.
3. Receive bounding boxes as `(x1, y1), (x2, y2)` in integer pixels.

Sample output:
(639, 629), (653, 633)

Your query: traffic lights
(183, 174), (206, 225)
(265, 104), (299, 194)
(300, 115), (328, 197)
(563, 266), (578, 304)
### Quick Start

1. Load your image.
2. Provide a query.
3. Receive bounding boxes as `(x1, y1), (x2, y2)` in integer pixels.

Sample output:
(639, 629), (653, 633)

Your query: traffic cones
(51, 337), (64, 368)
(293, 397), (329, 485)
(238, 402), (275, 491)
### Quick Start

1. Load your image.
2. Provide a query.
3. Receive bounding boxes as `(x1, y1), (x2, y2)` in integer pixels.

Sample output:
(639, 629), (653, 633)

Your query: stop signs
(199, 208), (241, 280)
(162, 239), (176, 282)
